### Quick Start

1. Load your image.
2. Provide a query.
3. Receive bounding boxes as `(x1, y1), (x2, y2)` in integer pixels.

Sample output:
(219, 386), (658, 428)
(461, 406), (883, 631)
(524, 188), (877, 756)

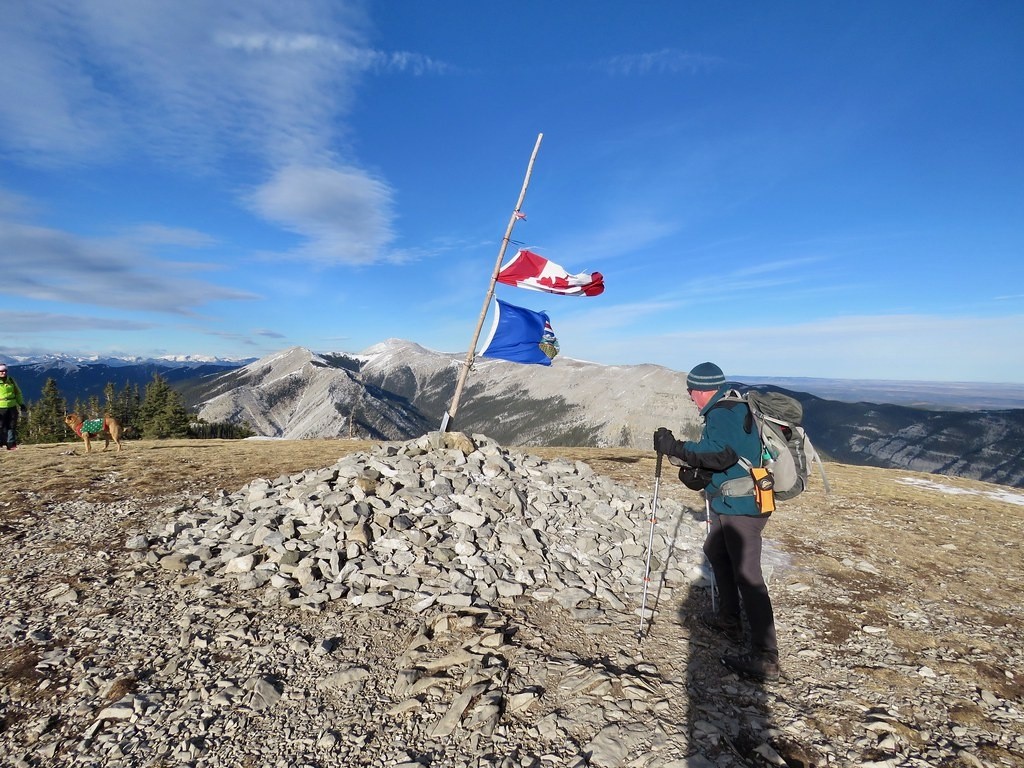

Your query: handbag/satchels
(678, 467), (713, 491)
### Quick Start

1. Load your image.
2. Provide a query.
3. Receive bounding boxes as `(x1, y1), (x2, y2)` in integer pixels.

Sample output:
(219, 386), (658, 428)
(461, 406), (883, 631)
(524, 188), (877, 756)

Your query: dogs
(63, 414), (131, 453)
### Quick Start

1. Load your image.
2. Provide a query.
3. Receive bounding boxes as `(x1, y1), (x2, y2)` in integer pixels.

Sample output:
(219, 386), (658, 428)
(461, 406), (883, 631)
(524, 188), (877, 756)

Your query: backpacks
(712, 388), (830, 502)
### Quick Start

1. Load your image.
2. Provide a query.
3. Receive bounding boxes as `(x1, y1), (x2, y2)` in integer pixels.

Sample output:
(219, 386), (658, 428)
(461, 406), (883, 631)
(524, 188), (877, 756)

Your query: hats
(686, 361), (725, 391)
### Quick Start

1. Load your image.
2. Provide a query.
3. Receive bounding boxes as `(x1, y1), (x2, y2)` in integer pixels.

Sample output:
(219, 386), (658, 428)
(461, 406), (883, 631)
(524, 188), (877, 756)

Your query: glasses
(0, 371), (7, 372)
(687, 388), (692, 395)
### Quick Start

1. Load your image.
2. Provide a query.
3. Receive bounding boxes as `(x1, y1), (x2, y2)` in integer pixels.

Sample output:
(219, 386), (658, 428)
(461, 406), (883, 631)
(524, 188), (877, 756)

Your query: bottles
(762, 435), (780, 465)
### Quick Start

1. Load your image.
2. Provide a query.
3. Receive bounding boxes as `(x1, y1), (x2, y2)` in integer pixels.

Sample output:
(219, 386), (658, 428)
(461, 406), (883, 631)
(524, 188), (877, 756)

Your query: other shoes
(720, 652), (780, 682)
(701, 612), (744, 642)
(7, 446), (18, 450)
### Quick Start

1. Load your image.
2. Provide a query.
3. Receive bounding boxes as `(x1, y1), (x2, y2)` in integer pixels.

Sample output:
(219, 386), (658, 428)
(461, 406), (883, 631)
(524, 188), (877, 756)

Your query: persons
(653, 362), (782, 687)
(0, 364), (28, 451)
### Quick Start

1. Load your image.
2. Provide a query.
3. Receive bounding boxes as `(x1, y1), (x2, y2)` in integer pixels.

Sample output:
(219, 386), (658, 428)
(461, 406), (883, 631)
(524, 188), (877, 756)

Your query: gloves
(654, 430), (676, 455)
(21, 405), (27, 412)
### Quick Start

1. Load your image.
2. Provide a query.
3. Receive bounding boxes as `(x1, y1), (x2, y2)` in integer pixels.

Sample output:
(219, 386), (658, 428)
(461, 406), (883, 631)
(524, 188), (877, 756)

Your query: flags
(494, 248), (604, 298)
(478, 293), (561, 368)
